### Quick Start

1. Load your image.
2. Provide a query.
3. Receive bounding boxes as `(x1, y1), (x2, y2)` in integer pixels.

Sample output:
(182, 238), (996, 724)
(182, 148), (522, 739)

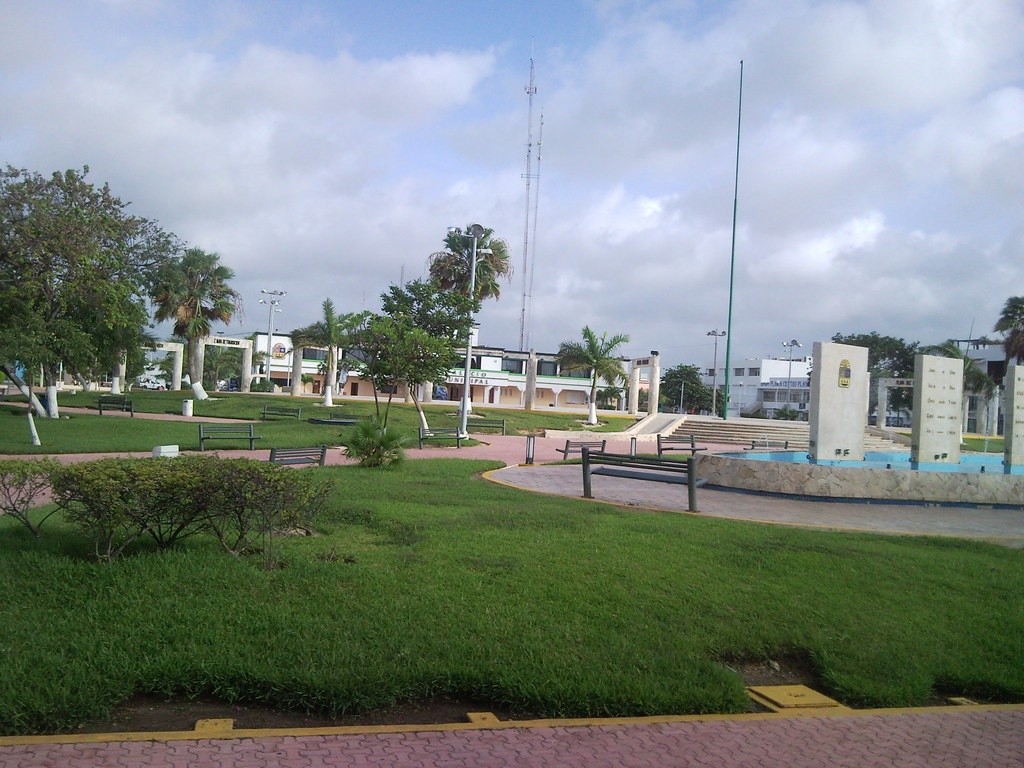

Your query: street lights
(783, 340), (802, 414)
(448, 224), (493, 440)
(260, 289), (286, 383)
(707, 329), (727, 417)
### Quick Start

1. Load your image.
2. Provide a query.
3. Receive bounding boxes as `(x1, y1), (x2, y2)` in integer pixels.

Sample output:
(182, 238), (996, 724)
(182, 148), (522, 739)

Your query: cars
(140, 379), (164, 390)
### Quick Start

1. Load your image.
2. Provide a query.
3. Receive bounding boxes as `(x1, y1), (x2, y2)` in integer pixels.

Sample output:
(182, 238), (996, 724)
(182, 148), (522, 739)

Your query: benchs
(743, 440), (789, 451)
(258, 444), (330, 466)
(556, 440), (607, 461)
(657, 435), (708, 458)
(418, 426), (465, 449)
(259, 406), (302, 419)
(94, 395), (134, 416)
(465, 418), (506, 435)
(196, 425), (262, 452)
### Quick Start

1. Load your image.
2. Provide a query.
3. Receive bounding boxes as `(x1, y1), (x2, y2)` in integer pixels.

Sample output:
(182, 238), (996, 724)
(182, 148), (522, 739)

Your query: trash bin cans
(183, 399), (194, 416)
